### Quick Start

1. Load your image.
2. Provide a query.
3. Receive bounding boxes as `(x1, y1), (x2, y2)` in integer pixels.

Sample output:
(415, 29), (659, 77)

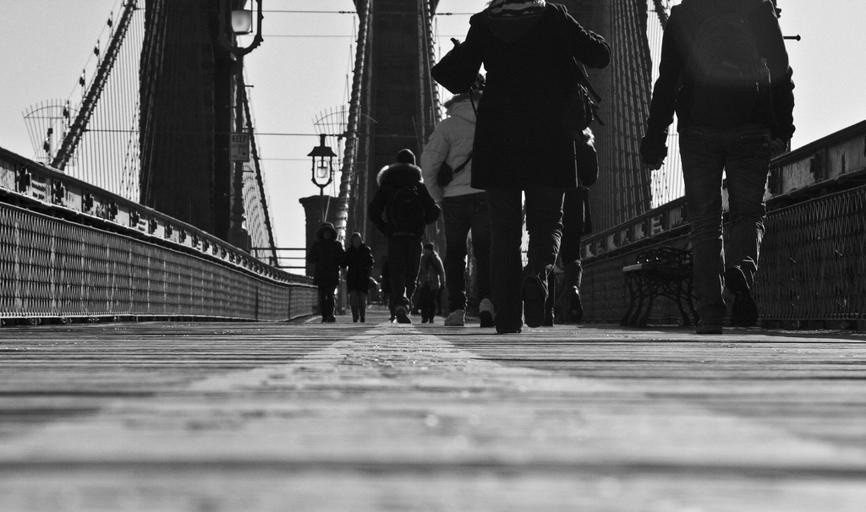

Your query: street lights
(218, 0), (264, 248)
(306, 134), (337, 222)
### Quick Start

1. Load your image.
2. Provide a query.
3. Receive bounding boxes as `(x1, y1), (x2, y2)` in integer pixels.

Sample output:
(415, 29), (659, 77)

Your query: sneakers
(698, 265), (758, 334)
(496, 275), (545, 332)
(443, 297), (495, 328)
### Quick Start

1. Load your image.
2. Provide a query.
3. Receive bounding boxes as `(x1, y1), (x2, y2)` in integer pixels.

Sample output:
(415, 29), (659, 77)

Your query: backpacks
(475, 3), (570, 139)
(676, 0), (773, 128)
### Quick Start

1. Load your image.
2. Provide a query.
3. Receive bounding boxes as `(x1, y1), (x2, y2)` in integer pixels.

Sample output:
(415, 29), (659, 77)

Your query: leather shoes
(568, 288), (583, 321)
(545, 308), (556, 327)
(320, 310), (434, 323)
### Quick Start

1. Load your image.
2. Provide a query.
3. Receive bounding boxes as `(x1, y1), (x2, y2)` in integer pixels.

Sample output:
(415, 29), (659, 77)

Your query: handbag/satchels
(563, 85), (594, 132)
(436, 163), (453, 186)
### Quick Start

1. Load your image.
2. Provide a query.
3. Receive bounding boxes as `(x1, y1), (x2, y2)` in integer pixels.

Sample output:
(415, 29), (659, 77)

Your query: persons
(638, 0), (798, 334)
(334, 257), (446, 317)
(418, 72), (500, 331)
(552, 128), (602, 328)
(427, 0), (612, 337)
(339, 231), (375, 323)
(305, 221), (346, 323)
(417, 241), (446, 324)
(366, 146), (442, 327)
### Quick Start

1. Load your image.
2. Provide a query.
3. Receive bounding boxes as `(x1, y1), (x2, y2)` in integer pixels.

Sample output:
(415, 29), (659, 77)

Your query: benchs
(620, 233), (699, 329)
